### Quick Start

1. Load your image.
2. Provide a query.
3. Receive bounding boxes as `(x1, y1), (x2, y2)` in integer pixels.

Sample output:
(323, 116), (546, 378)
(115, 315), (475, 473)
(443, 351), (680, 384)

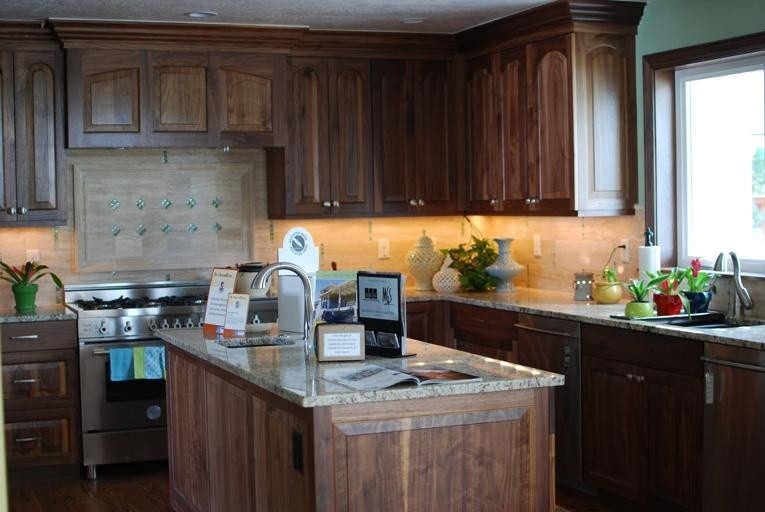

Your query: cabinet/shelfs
(0, 319), (82, 484)
(580, 321), (703, 511)
(66, 45), (287, 151)
(0, 39), (70, 229)
(264, 56), (376, 220)
(404, 300), (439, 347)
(451, 303), (519, 368)
(369, 59), (470, 218)
(503, 21), (640, 218)
(471, 50), (504, 217)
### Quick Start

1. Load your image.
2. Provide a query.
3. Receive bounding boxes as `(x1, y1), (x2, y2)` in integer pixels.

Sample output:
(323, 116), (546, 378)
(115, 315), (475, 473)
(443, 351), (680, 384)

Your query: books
(318, 365), (487, 395)
(354, 269), (408, 359)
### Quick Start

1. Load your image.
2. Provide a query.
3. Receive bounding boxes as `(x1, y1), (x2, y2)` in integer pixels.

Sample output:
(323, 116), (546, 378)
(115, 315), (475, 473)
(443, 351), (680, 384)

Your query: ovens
(79, 342), (168, 480)
(516, 312), (585, 498)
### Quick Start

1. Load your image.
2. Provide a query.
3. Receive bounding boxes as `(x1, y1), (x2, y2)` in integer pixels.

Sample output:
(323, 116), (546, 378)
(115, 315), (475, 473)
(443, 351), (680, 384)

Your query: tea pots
(229, 261), (273, 298)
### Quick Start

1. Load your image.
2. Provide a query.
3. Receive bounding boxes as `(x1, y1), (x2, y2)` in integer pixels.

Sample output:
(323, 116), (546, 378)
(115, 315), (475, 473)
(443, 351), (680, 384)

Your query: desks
(151, 326), (569, 512)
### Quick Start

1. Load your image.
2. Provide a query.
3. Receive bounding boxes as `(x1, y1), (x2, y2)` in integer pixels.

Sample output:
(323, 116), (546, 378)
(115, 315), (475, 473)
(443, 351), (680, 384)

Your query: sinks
(636, 313), (765, 329)
(214, 336), (296, 348)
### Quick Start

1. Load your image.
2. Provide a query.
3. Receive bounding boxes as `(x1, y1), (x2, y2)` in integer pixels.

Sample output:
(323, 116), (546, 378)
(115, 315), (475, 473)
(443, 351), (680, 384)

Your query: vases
(678, 287), (713, 314)
(10, 283), (38, 312)
(485, 237), (529, 294)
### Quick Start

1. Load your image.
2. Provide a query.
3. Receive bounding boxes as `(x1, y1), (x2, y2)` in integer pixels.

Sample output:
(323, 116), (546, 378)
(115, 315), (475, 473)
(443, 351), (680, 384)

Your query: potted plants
(592, 266), (624, 305)
(621, 274), (671, 320)
(645, 266), (693, 316)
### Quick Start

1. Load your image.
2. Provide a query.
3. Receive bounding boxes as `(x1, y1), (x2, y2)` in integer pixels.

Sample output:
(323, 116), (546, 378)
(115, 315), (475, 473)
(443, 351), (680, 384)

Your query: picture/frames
(313, 322), (367, 363)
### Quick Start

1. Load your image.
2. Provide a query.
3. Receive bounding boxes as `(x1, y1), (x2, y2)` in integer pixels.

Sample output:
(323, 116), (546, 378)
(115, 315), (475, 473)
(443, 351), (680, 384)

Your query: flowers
(0, 257), (67, 291)
(682, 254), (724, 296)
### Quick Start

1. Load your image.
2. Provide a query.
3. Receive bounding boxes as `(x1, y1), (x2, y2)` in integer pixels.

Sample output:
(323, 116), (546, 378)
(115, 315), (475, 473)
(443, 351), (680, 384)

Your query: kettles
(572, 270), (594, 301)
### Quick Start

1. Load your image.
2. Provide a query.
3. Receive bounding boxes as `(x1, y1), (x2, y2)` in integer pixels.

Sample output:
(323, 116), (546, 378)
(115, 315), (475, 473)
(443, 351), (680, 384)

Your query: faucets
(250, 262), (313, 341)
(728, 252), (754, 317)
(303, 342), (314, 397)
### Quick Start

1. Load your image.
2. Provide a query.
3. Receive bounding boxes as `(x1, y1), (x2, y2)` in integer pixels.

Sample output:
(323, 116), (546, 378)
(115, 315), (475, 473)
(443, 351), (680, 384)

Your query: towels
(133, 342), (169, 382)
(109, 346), (136, 382)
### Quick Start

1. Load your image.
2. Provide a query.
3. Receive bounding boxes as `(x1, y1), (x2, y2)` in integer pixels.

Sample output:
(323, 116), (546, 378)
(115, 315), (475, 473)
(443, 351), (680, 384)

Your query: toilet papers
(638, 245), (660, 301)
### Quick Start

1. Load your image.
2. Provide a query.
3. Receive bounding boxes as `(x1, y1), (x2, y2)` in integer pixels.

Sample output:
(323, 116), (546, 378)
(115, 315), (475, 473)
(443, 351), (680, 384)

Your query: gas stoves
(67, 294), (279, 342)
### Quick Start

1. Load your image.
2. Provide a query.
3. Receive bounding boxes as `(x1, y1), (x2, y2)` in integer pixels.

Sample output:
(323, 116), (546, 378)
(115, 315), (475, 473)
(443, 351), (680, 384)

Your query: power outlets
(532, 233), (545, 258)
(619, 238), (632, 264)
(377, 237), (391, 262)
